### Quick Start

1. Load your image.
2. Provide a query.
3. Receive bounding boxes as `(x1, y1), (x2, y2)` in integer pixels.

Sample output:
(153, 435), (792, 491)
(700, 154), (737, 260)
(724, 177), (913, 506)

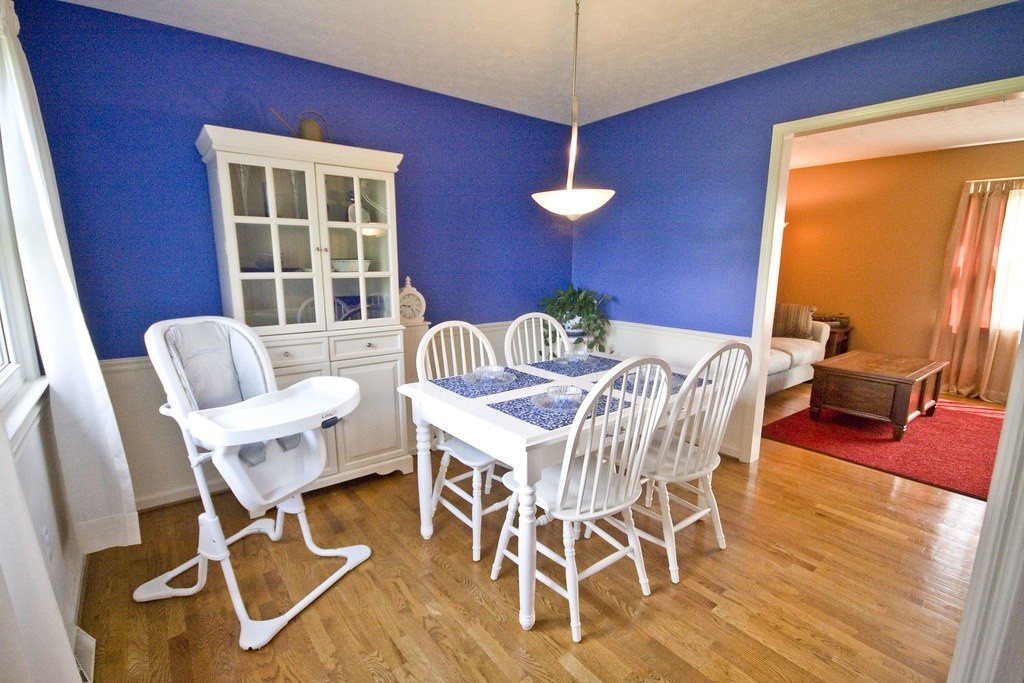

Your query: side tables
(813, 320), (854, 360)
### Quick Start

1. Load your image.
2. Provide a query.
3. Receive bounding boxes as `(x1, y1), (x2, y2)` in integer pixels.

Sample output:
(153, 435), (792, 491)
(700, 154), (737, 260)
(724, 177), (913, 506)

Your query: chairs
(585, 340), (753, 584)
(486, 354), (680, 644)
(134, 316), (372, 651)
(504, 312), (572, 366)
(414, 320), (512, 561)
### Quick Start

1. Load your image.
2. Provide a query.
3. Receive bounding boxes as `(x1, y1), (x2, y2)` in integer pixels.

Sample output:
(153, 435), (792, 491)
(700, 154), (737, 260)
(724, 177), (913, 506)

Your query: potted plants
(537, 281), (615, 360)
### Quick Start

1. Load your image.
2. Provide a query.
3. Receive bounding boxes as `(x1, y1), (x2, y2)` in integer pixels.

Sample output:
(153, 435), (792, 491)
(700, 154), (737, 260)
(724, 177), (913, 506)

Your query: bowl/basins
(547, 386), (582, 402)
(564, 350), (589, 363)
(474, 365), (504, 381)
(331, 259), (371, 272)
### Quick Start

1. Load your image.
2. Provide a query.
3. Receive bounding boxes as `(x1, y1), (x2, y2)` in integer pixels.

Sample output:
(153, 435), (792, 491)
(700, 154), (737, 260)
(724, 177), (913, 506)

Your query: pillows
(772, 303), (817, 340)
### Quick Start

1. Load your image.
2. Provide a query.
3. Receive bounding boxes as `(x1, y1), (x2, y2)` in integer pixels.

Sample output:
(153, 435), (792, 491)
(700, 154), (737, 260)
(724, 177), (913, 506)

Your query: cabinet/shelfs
(194, 124), (415, 520)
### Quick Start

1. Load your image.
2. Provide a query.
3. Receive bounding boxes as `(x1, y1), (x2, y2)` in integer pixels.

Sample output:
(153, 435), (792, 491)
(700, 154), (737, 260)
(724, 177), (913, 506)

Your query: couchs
(766, 321), (831, 395)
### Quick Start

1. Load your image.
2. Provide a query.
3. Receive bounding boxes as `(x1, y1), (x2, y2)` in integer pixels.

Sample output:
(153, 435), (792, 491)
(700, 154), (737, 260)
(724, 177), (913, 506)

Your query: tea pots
(269, 107), (331, 143)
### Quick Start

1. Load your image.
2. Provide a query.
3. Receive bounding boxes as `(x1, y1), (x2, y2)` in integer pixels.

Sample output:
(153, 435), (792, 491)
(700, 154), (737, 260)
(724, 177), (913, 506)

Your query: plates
(463, 372), (516, 386)
(531, 393), (587, 414)
(552, 357), (602, 367)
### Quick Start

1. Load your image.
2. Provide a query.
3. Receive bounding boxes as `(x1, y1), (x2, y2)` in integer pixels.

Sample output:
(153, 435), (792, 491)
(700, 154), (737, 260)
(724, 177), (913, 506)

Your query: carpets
(762, 401), (1006, 502)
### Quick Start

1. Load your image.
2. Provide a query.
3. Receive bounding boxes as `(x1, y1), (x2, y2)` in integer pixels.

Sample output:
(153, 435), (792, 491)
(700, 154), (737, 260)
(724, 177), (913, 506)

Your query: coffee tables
(810, 349), (950, 441)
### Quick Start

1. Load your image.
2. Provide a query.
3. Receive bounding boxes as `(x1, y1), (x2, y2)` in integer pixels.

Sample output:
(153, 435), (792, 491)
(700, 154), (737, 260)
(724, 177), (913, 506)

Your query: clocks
(399, 290), (426, 324)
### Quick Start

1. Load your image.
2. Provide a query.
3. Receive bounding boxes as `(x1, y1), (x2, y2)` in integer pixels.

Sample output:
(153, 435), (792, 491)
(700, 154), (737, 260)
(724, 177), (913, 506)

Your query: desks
(395, 352), (722, 631)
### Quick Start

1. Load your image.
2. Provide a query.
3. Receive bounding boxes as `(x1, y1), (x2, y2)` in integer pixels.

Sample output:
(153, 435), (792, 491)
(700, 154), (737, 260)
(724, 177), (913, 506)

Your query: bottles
(346, 190), (372, 223)
(811, 312), (849, 328)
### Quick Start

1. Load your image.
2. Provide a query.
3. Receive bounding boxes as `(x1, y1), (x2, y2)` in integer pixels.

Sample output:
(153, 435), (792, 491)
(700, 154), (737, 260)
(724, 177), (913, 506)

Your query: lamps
(530, 0), (616, 221)
